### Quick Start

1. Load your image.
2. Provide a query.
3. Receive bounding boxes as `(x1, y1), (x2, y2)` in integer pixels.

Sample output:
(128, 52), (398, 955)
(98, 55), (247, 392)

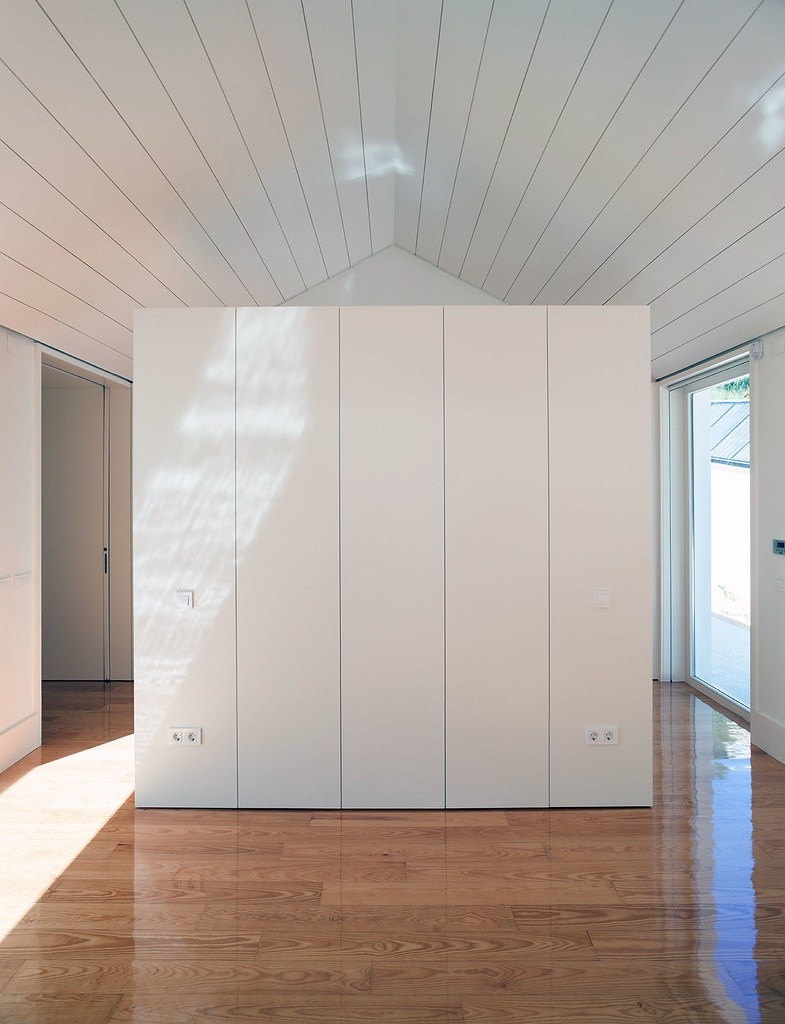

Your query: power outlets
(169, 727), (202, 747)
(584, 725), (618, 745)
